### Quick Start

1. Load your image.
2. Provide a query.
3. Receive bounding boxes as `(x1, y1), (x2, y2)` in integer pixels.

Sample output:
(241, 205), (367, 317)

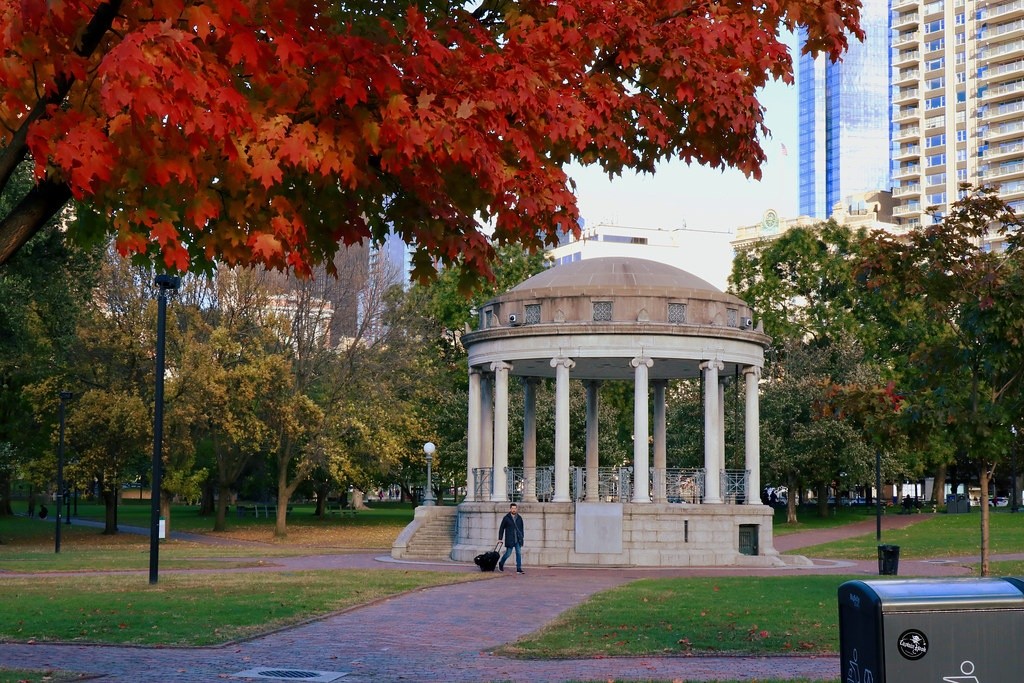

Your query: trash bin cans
(838, 575), (1024, 683)
(946, 494), (970, 513)
(878, 545), (900, 576)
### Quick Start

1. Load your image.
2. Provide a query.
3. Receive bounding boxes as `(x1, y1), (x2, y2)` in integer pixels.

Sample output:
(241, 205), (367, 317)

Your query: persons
(901, 494), (913, 515)
(994, 496), (999, 507)
(498, 503), (525, 575)
(379, 489), (400, 499)
(763, 490), (778, 503)
(28, 495), (48, 520)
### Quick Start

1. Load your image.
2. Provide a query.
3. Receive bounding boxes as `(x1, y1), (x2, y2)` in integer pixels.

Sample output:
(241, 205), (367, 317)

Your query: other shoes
(516, 568), (525, 574)
(498, 562), (503, 572)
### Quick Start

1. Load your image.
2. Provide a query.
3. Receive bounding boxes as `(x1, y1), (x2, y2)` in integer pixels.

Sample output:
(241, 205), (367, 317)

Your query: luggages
(474, 542), (503, 572)
